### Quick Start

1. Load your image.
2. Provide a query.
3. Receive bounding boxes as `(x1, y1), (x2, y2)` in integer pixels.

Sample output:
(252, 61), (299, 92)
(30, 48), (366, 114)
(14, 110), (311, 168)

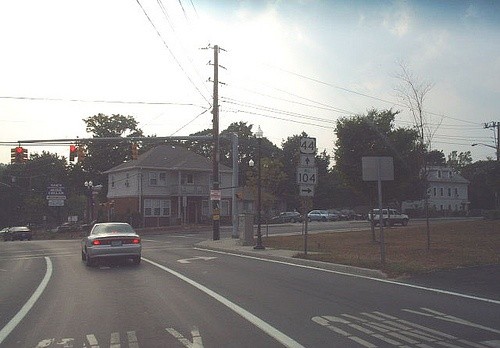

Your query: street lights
(248, 125), (269, 249)
(84, 180), (94, 225)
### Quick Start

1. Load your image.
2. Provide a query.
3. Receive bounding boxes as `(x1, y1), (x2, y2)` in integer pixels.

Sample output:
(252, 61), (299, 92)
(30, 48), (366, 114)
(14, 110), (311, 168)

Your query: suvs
(368, 207), (409, 227)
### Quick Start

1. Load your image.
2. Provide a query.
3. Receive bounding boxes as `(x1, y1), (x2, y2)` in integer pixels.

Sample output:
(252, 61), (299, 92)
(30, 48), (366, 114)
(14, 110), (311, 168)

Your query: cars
(0, 227), (11, 234)
(81, 221), (142, 267)
(4, 226), (32, 241)
(308, 209), (337, 222)
(271, 211), (302, 224)
(327, 208), (363, 221)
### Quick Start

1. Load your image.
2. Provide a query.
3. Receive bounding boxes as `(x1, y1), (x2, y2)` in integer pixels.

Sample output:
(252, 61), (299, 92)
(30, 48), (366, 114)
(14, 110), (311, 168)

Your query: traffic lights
(10, 148), (16, 164)
(22, 148), (28, 163)
(16, 147), (23, 163)
(69, 145), (75, 161)
